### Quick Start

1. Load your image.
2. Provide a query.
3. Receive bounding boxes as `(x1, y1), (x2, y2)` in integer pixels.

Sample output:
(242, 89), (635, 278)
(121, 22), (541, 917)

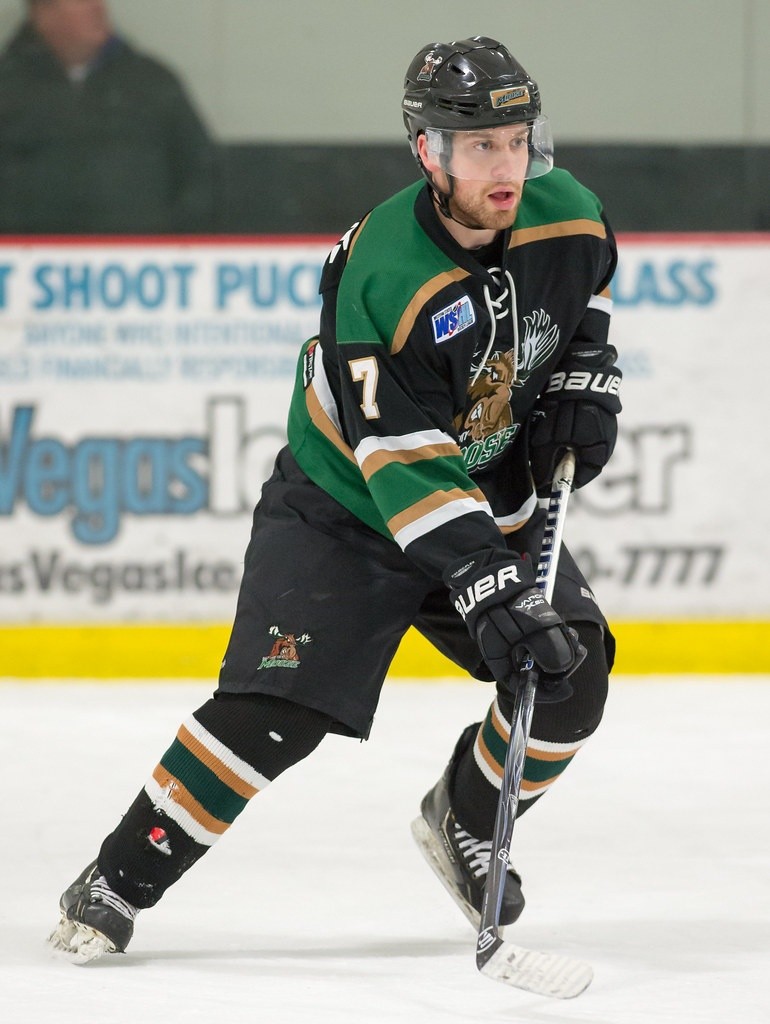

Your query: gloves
(527, 308), (621, 498)
(441, 547), (588, 705)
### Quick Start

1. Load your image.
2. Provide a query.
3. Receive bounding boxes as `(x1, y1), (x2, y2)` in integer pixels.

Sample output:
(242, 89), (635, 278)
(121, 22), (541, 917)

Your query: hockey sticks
(474, 446), (592, 999)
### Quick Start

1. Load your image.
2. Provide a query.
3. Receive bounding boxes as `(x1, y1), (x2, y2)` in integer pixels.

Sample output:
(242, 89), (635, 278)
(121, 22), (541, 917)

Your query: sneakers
(45, 856), (138, 966)
(410, 757), (525, 942)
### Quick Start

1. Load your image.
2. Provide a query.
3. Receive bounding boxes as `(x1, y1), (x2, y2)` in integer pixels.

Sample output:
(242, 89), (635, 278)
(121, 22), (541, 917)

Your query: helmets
(401, 37), (542, 158)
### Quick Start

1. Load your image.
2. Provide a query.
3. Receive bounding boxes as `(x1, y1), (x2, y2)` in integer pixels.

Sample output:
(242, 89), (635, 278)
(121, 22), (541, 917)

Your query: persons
(0, 0), (217, 235)
(46, 37), (622, 968)
